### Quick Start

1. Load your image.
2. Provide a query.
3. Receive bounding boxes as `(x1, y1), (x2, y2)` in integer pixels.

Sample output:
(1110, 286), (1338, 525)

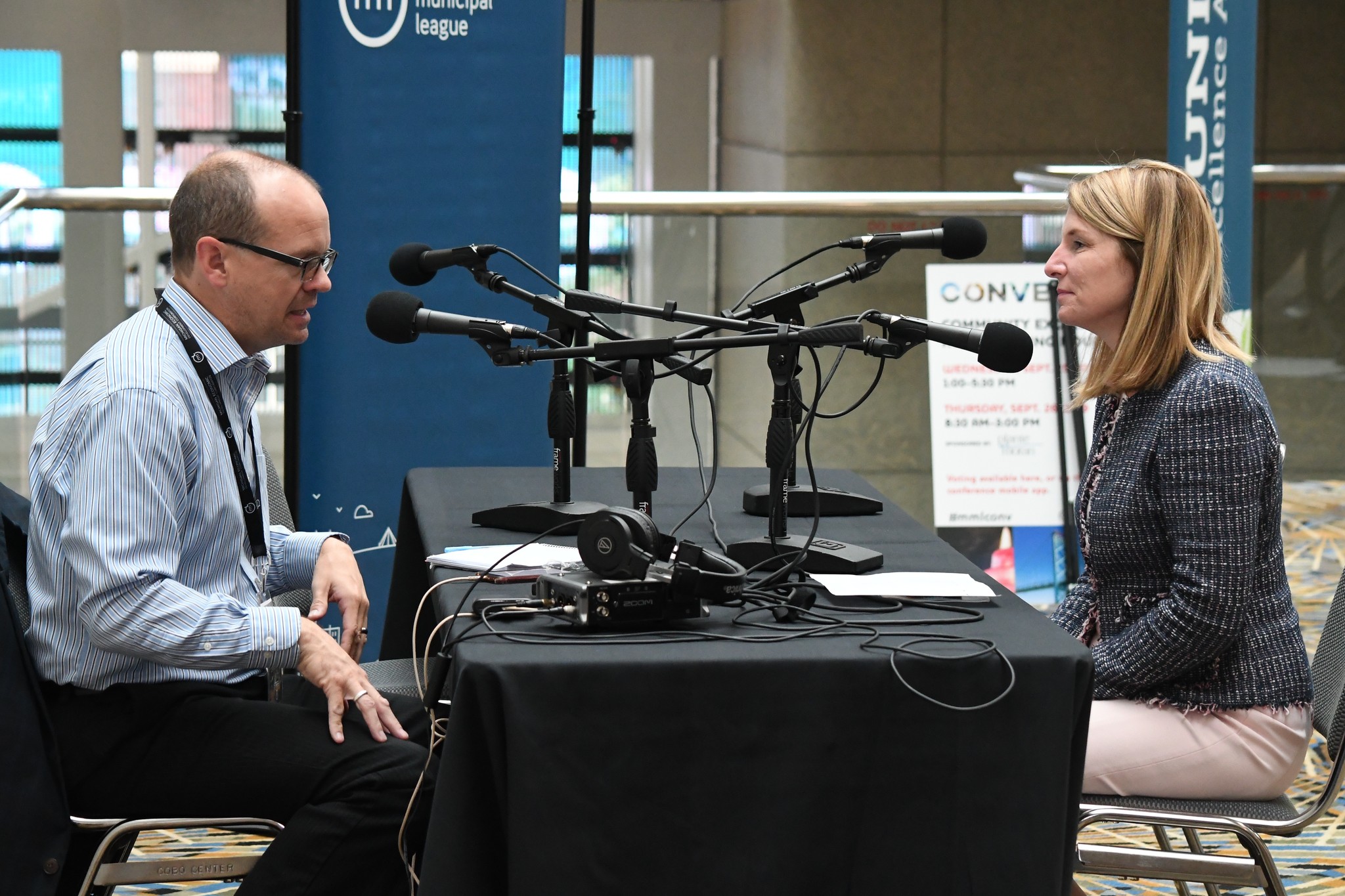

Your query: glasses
(217, 236), (338, 283)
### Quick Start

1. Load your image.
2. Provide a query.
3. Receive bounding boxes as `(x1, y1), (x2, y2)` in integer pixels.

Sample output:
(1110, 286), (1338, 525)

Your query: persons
(1042, 156), (1318, 802)
(21, 144), (442, 896)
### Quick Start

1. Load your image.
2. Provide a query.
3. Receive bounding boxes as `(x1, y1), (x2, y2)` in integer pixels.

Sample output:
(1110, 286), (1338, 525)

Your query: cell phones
(476, 567), (572, 585)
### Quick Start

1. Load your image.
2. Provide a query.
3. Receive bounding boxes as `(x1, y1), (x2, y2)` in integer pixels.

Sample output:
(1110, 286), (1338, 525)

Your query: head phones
(577, 506), (747, 602)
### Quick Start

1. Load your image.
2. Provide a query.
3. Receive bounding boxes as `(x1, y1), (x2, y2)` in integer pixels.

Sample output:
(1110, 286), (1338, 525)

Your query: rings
(353, 690), (368, 702)
(360, 627), (368, 635)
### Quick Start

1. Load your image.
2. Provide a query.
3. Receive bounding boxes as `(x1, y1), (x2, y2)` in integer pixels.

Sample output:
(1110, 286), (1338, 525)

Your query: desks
(378, 467), (1095, 895)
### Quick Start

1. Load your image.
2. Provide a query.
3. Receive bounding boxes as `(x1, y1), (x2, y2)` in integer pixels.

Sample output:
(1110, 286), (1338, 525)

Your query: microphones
(366, 290), (539, 344)
(838, 216), (988, 260)
(865, 312), (1034, 373)
(389, 242), (498, 286)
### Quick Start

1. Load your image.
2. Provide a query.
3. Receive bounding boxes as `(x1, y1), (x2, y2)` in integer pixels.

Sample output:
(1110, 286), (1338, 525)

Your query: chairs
(1067, 569), (1345, 894)
(0, 481), (292, 896)
